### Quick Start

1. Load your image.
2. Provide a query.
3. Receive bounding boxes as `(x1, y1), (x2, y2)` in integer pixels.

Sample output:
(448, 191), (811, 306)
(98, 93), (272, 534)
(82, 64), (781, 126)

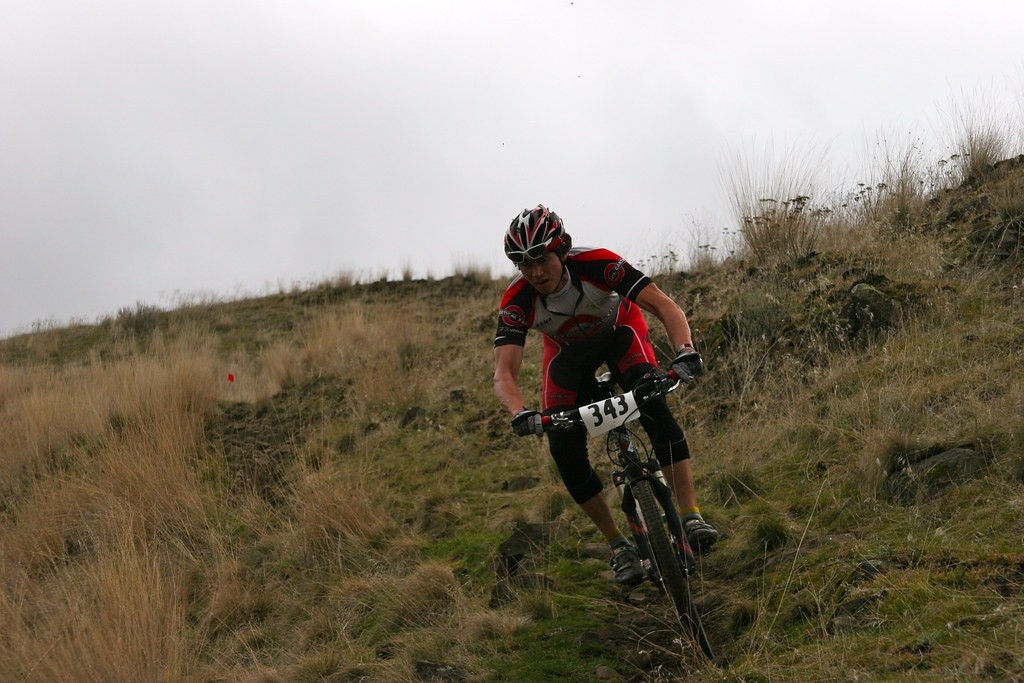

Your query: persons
(491, 203), (720, 589)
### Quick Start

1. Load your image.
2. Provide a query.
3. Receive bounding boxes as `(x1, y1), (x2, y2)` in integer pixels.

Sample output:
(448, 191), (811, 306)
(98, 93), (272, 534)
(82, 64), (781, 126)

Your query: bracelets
(676, 343), (694, 352)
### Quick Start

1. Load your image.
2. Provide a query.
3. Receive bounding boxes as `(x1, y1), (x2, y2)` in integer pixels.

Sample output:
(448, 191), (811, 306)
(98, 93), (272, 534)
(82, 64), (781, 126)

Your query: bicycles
(514, 357), (718, 666)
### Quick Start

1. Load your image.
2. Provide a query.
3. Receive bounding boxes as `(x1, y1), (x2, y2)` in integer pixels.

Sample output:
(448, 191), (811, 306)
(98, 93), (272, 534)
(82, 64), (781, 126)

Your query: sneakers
(680, 512), (717, 551)
(609, 546), (645, 583)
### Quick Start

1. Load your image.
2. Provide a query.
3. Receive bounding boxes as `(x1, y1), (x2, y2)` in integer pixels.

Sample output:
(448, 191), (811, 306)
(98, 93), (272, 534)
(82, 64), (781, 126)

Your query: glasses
(507, 237), (554, 263)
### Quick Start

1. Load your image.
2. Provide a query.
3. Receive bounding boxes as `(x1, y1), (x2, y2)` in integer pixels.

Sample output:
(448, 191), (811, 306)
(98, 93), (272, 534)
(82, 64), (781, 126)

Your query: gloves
(670, 343), (703, 384)
(510, 407), (544, 438)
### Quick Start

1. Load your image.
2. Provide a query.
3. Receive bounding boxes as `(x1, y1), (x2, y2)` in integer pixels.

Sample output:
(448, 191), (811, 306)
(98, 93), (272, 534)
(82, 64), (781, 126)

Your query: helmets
(504, 204), (565, 264)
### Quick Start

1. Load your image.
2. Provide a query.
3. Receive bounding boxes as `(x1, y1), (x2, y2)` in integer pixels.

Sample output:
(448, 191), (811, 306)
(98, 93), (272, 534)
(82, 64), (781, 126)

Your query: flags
(228, 373), (234, 382)
